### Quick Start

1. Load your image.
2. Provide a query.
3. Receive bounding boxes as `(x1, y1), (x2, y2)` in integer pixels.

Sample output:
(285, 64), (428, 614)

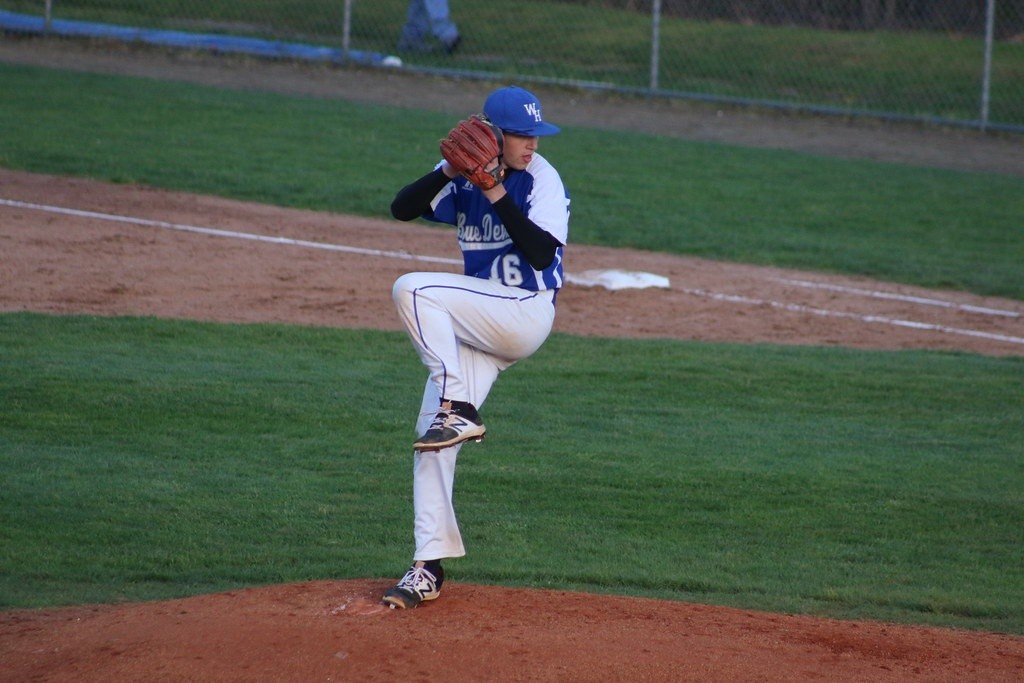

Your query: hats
(483, 85), (561, 136)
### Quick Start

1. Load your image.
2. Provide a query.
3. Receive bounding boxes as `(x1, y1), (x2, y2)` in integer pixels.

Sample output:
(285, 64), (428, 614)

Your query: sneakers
(411, 399), (486, 450)
(381, 561), (444, 610)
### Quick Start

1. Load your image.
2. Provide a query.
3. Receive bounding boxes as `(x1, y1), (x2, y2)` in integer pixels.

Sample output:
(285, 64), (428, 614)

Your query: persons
(382, 86), (570, 609)
(397, 0), (461, 56)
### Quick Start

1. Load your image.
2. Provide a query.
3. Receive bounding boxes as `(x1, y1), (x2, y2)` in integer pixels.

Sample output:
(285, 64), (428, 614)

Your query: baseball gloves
(438, 113), (505, 190)
